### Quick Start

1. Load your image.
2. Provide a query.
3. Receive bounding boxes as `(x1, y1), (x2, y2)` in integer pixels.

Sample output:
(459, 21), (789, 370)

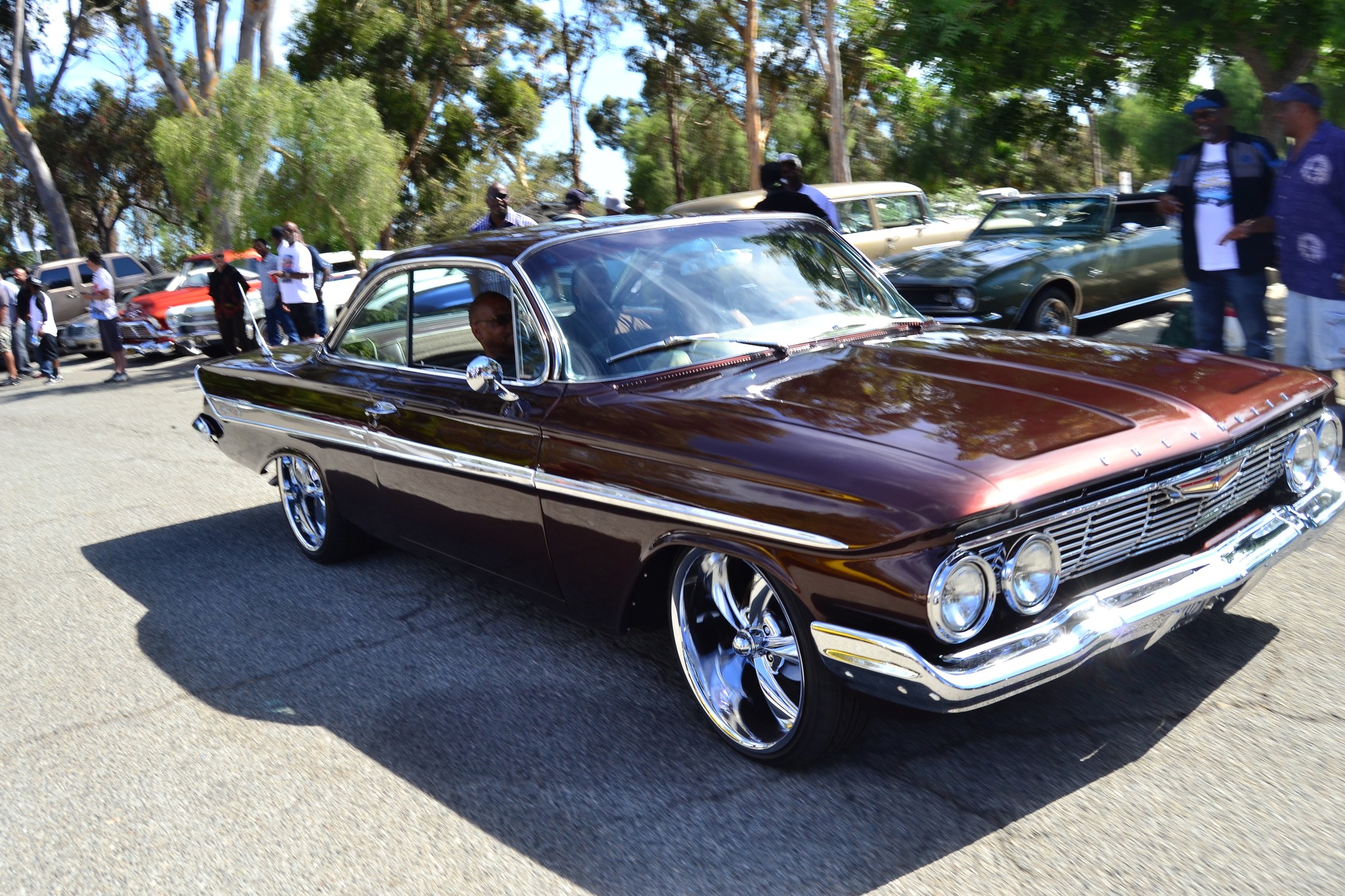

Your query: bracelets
(556, 294), (567, 302)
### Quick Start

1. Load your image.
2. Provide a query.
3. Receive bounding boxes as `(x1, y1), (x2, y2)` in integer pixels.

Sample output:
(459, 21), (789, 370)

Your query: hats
(604, 196), (632, 213)
(272, 226), (286, 238)
(1262, 84), (1324, 107)
(1182, 95), (1225, 114)
(26, 276), (44, 287)
(565, 187), (595, 203)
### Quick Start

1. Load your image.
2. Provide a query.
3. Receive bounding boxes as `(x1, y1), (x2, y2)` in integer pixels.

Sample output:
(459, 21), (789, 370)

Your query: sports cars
(193, 213), (1345, 769)
(867, 190), (1196, 336)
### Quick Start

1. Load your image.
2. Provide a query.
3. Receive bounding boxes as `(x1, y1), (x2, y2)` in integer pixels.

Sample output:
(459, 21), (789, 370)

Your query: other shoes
(0, 375), (21, 387)
(104, 371), (129, 383)
(43, 374), (64, 386)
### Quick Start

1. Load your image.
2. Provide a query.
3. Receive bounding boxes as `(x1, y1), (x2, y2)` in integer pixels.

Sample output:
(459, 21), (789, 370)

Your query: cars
(662, 182), (1035, 270)
(57, 272), (179, 360)
(115, 245), (476, 360)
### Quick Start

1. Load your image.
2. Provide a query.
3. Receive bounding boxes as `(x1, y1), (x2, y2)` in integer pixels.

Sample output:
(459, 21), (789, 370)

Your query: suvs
(1, 253), (158, 324)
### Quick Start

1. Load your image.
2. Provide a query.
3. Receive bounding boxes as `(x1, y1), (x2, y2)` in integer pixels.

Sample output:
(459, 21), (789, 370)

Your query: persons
(209, 251), (250, 353)
(601, 194), (633, 281)
(0, 267), (64, 386)
(468, 289), (567, 381)
(253, 221), (333, 341)
(1155, 89), (1281, 363)
(78, 251), (131, 383)
(1218, 83), (1345, 409)
(558, 259), (664, 377)
(549, 188), (595, 257)
(455, 183), (569, 305)
(658, 251), (752, 335)
(754, 152), (841, 271)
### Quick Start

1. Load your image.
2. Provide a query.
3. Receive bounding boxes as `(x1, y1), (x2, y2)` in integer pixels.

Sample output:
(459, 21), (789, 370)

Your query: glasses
(474, 313), (512, 325)
(487, 193), (509, 199)
(213, 254), (224, 260)
